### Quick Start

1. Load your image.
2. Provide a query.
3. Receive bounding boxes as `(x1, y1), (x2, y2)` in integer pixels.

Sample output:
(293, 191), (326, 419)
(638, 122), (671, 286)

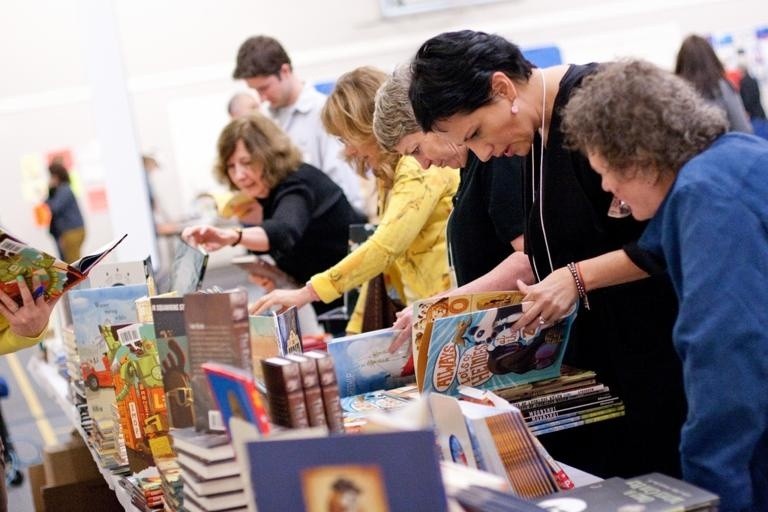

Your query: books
(0, 223), (130, 307)
(34, 253), (720, 512)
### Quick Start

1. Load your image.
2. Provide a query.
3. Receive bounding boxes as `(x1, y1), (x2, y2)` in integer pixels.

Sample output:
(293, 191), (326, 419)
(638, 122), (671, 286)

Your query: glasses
(607, 179), (632, 218)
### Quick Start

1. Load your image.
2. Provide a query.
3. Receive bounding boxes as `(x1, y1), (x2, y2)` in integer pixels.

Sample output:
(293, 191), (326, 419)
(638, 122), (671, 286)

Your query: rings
(12, 304), (21, 315)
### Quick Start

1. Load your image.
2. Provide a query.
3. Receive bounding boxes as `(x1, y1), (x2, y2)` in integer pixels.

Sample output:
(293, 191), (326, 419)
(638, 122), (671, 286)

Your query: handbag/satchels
(361, 267), (406, 333)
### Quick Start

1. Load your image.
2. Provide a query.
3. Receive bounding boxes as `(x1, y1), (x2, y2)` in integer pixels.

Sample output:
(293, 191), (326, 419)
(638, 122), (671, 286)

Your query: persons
(182, 29), (767, 512)
(0, 271), (55, 358)
(45, 162), (87, 262)
